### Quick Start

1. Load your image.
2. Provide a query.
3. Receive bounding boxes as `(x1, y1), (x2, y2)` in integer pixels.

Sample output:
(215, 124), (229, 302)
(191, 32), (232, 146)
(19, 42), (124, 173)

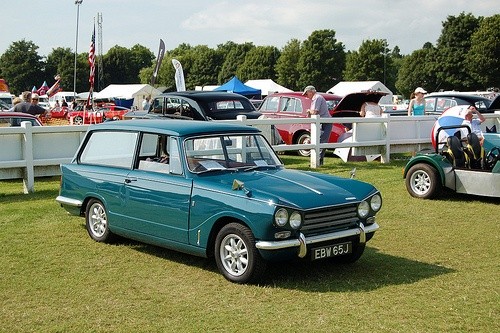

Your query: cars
(55, 118), (383, 284)
(0, 90), (500, 161)
(402, 124), (500, 200)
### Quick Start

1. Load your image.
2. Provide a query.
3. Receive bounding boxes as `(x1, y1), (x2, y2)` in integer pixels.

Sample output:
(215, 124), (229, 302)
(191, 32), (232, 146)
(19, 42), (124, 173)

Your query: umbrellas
(431, 105), (471, 153)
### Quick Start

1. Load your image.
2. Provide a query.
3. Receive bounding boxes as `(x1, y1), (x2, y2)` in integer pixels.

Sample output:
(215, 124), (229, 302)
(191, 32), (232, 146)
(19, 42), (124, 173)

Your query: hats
(302, 85), (315, 95)
(415, 87), (427, 93)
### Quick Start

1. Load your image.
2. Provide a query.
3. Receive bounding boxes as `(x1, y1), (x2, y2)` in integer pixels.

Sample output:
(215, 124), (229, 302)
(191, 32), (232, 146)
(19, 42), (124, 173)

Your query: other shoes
(308, 158), (323, 166)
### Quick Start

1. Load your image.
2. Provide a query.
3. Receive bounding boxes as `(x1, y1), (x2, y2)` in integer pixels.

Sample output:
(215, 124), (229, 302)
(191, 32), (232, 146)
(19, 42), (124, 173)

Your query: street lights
(74, 0), (83, 102)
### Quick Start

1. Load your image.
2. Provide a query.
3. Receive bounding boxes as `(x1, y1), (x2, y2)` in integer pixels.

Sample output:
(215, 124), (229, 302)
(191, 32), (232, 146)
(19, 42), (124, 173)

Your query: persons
(408, 86), (426, 116)
(302, 85), (333, 165)
(0, 91), (48, 126)
(55, 100), (60, 111)
(326, 92), (337, 108)
(62, 100), (68, 108)
(359, 101), (383, 118)
(71, 99), (78, 110)
(460, 106), (487, 140)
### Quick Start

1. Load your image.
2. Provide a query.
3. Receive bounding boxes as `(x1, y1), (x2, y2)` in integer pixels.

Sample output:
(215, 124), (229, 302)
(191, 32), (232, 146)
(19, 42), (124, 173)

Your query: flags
(153, 39), (165, 77)
(172, 59), (186, 92)
(89, 31), (95, 94)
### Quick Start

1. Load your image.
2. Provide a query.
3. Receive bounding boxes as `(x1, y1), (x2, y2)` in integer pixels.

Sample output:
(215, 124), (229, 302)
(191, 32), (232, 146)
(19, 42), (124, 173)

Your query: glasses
(32, 98), (38, 102)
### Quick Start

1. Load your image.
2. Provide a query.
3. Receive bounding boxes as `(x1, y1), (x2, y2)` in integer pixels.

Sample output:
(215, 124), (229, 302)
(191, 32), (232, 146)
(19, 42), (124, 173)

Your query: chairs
(443, 133), (486, 169)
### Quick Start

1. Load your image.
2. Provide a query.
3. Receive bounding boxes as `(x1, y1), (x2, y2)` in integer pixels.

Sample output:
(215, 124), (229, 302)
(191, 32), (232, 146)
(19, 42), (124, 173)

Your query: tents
(94, 84), (163, 111)
(326, 81), (393, 96)
(142, 97), (151, 112)
(212, 76), (262, 100)
(243, 79), (294, 96)
(51, 91), (99, 100)
